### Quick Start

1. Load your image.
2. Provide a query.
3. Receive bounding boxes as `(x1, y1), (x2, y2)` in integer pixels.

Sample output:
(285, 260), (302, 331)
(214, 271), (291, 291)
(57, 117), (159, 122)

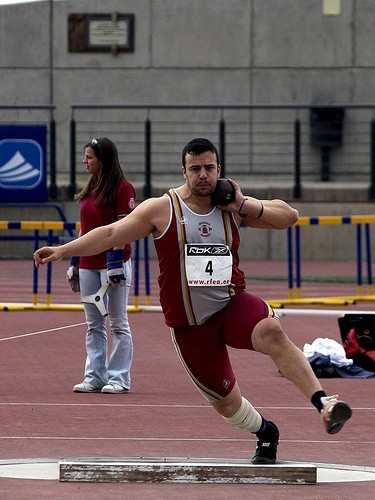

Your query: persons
(32, 137), (352, 464)
(67, 138), (136, 394)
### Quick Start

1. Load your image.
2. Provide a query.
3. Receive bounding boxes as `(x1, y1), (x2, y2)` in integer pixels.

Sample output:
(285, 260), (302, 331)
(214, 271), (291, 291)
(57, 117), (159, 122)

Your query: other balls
(209, 179), (236, 207)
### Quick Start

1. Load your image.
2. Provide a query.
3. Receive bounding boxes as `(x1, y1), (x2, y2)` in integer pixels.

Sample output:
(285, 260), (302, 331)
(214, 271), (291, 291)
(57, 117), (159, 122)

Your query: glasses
(90, 138), (98, 149)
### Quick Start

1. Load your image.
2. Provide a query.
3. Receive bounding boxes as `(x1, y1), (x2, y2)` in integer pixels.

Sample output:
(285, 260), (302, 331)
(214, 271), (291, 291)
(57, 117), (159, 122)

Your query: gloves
(106, 249), (125, 288)
(66, 256), (80, 292)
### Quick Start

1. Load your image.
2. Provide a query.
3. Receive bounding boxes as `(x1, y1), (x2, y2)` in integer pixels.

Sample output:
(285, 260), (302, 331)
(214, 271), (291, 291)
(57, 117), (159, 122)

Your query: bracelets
(237, 199), (249, 218)
(256, 201), (264, 221)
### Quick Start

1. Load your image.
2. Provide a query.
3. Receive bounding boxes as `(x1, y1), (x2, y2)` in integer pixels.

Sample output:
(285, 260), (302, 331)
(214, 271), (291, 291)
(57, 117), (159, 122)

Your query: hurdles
(0, 214), (375, 312)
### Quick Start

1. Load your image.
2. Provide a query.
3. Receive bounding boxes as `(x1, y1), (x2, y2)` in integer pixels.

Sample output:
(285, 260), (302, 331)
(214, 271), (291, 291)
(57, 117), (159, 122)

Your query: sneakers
(73, 382), (102, 393)
(251, 421), (280, 464)
(102, 383), (127, 393)
(320, 395), (353, 435)
